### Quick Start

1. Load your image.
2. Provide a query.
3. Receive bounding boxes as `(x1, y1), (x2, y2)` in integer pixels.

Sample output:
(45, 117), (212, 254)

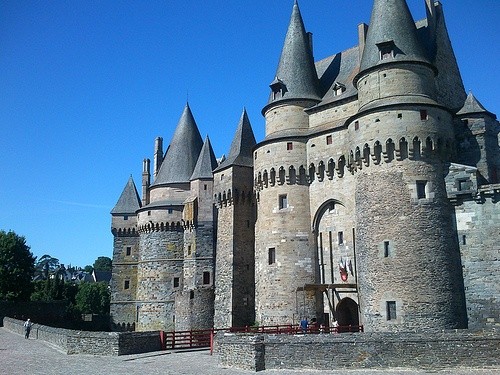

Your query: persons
(331, 316), (339, 333)
(298, 316), (309, 334)
(24, 318), (32, 339)
